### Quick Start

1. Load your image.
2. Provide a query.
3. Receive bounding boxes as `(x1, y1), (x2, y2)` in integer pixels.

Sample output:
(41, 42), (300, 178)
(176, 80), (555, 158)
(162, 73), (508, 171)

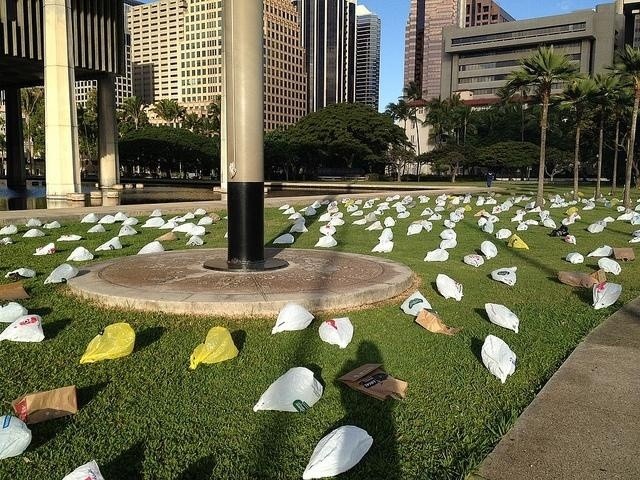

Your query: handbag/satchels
(339, 363), (408, 401)
(12, 384), (77, 425)
(415, 307), (463, 335)
(612, 247), (636, 263)
(0, 282), (32, 301)
(590, 268), (607, 283)
